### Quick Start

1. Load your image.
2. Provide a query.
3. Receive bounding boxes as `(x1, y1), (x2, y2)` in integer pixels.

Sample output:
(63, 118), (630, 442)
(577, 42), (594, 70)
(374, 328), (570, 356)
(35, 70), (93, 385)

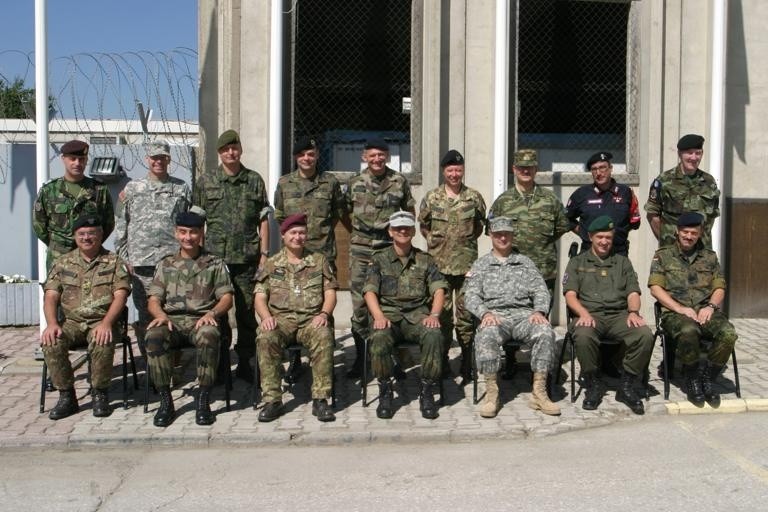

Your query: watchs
(706, 303), (717, 310)
(319, 311), (330, 317)
(629, 310), (640, 314)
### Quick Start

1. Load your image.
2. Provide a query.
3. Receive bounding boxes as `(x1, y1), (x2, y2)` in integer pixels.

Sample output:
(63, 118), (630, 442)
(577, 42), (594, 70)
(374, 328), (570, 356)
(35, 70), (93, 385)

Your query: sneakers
(389, 212), (416, 229)
(235, 355), (260, 386)
(280, 214), (308, 236)
(92, 389), (111, 416)
(311, 399), (337, 421)
(258, 400), (286, 422)
(657, 360), (664, 380)
(218, 356), (235, 384)
(45, 377), (57, 392)
(49, 389), (81, 419)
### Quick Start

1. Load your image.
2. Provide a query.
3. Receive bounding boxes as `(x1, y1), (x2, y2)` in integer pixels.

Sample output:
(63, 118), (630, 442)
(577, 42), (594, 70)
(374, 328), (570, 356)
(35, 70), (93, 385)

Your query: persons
(362, 212), (448, 418)
(649, 214), (736, 402)
(145, 212), (233, 425)
(419, 150), (486, 376)
(342, 137), (416, 378)
(34, 140), (116, 392)
(116, 139), (191, 375)
(487, 149), (568, 378)
(254, 215), (338, 421)
(193, 130), (268, 382)
(40, 215), (131, 419)
(565, 217), (654, 413)
(465, 217), (561, 418)
(567, 152), (641, 378)
(274, 136), (343, 382)
(643, 134), (720, 378)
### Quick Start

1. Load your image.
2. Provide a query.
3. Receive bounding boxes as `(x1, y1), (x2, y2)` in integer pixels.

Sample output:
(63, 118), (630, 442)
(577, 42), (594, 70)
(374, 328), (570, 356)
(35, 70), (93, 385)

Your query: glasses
(591, 165), (612, 174)
(75, 229), (101, 239)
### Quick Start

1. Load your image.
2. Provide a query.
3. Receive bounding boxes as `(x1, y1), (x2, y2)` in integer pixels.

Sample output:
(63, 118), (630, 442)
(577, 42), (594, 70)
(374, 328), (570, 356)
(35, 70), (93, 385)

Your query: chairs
(143, 311), (232, 413)
(41, 306), (137, 413)
(651, 301), (741, 400)
(468, 318), (552, 404)
(253, 315), (337, 409)
(363, 334), (445, 407)
(556, 304), (649, 403)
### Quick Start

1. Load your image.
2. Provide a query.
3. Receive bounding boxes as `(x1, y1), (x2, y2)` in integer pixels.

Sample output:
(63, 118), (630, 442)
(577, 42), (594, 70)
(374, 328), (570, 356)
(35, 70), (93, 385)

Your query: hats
(292, 136), (320, 156)
(61, 141), (89, 157)
(440, 150), (464, 168)
(73, 214), (103, 233)
(365, 136), (390, 151)
(587, 215), (616, 234)
(216, 130), (241, 152)
(175, 212), (204, 227)
(677, 134), (705, 152)
(147, 140), (171, 157)
(514, 150), (539, 168)
(586, 151), (614, 170)
(488, 217), (515, 233)
(677, 212), (706, 228)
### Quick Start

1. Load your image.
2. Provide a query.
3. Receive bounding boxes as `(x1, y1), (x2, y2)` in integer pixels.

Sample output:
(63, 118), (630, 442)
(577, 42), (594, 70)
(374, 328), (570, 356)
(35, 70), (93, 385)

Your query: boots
(614, 370), (645, 414)
(500, 353), (521, 380)
(419, 382), (438, 419)
(195, 386), (217, 425)
(479, 374), (503, 417)
(394, 353), (408, 380)
(154, 386), (176, 426)
(342, 340), (368, 380)
(527, 370), (561, 416)
(582, 370), (607, 409)
(705, 363), (721, 405)
(443, 353), (456, 381)
(688, 363), (705, 404)
(376, 378), (393, 419)
(458, 349), (472, 386)
(284, 349), (304, 382)
